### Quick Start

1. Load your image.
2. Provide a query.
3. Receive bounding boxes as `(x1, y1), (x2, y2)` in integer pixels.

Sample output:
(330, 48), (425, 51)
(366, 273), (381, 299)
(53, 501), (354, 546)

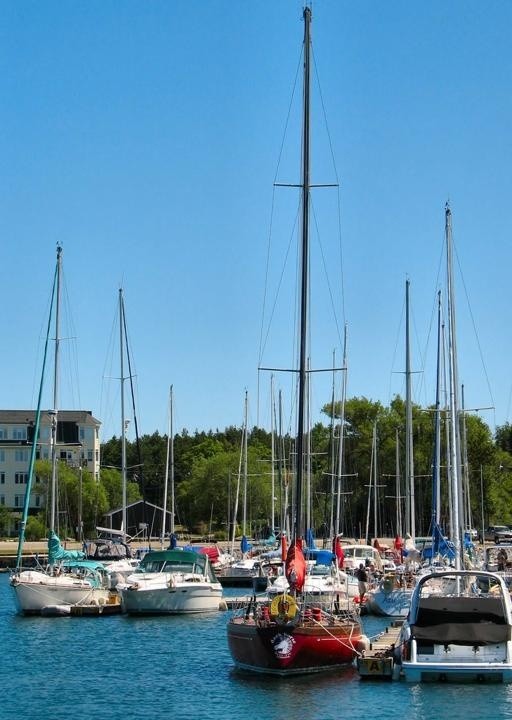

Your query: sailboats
(127, 386), (223, 617)
(107, 286), (145, 591)
(10, 240), (125, 617)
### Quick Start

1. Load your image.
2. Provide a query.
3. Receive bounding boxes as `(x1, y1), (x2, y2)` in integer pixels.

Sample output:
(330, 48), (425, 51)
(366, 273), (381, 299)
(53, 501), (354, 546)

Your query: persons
(365, 565), (383, 588)
(82, 539), (87, 552)
(357, 564), (367, 606)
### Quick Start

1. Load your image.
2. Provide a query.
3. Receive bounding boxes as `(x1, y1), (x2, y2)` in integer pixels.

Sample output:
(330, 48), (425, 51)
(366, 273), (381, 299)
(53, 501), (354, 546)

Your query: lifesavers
(271, 595), (296, 617)
(379, 580), (394, 594)
